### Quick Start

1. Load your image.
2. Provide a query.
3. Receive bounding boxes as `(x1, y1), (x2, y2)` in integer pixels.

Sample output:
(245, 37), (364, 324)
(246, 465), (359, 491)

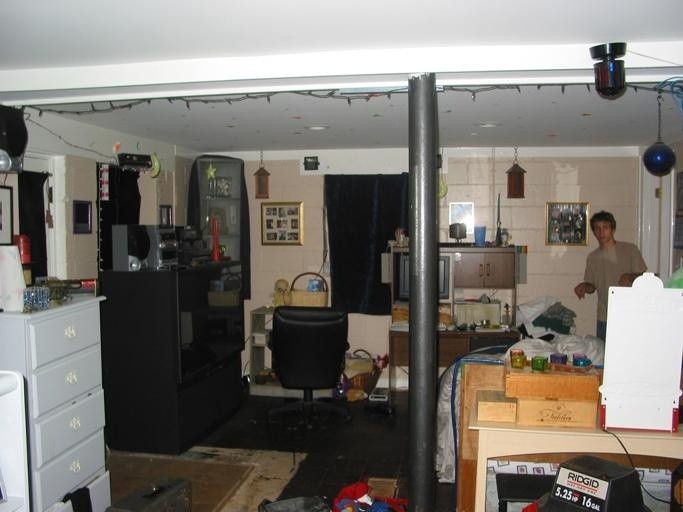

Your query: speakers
(111, 224), (151, 271)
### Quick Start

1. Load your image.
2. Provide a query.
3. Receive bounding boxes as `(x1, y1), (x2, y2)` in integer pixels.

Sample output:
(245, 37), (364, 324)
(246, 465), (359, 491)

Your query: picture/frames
(72, 200), (93, 234)
(544, 201), (591, 247)
(0, 185), (14, 246)
(160, 205), (174, 228)
(260, 202), (304, 246)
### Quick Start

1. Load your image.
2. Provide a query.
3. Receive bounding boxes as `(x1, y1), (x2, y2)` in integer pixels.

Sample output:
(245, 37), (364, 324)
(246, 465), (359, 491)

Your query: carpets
(109, 452), (255, 511)
(190, 445), (309, 512)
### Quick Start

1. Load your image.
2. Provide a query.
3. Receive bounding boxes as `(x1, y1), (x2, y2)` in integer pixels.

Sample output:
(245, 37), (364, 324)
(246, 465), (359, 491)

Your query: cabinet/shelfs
(1, 294), (108, 512)
(196, 158), (244, 261)
(249, 306), (275, 383)
(452, 247), (517, 290)
(95, 261), (250, 454)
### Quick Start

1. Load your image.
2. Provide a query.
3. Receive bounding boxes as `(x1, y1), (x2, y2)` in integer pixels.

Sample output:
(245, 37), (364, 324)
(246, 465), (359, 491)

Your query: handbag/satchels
(333, 349), (382, 400)
(290, 272), (328, 306)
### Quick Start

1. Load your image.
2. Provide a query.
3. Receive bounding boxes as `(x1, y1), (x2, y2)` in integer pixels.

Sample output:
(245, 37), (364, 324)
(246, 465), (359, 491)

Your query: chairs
(265, 305), (353, 434)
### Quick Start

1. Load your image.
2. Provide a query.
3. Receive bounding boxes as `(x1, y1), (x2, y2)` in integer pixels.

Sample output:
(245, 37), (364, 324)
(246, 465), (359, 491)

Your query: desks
(389, 328), (520, 415)
(468, 402), (683, 512)
(455, 351), (601, 512)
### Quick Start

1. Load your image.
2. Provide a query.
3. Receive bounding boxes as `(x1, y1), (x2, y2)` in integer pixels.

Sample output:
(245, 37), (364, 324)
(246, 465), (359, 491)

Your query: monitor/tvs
(399, 256), (449, 299)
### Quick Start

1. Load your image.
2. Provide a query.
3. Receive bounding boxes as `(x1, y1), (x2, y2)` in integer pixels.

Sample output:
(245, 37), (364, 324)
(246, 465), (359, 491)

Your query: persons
(574, 210), (648, 341)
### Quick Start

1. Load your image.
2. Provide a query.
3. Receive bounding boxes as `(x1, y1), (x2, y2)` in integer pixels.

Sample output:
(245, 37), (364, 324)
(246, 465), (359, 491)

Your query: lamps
(643, 93), (675, 176)
(506, 145), (525, 200)
(590, 42), (627, 100)
(253, 148), (270, 199)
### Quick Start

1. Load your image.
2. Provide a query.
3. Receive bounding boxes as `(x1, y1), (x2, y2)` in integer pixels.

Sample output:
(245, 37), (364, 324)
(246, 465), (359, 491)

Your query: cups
(475, 226), (486, 248)
(23, 286), (48, 310)
